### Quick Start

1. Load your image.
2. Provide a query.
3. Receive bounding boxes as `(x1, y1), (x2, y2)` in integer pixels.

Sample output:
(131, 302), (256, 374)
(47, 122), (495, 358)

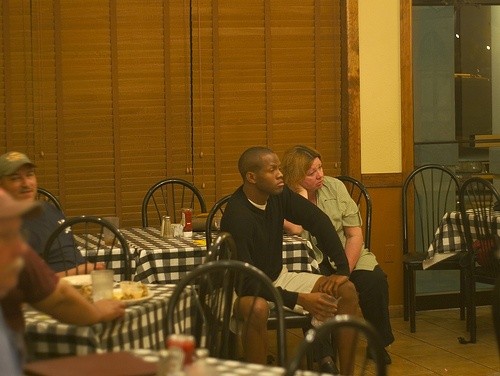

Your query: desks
(422, 206), (500, 330)
(75, 226), (324, 284)
(23, 282), (197, 357)
(22, 348), (335, 376)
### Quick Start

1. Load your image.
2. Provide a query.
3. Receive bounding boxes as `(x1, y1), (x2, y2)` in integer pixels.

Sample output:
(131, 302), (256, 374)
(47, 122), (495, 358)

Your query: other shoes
(366, 347), (391, 364)
(321, 355), (339, 374)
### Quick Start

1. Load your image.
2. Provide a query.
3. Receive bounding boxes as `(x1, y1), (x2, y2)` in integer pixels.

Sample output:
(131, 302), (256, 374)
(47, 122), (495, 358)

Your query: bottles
(161, 216), (173, 239)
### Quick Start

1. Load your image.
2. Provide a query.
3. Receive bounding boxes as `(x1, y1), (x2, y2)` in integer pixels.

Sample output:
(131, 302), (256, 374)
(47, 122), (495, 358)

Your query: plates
(113, 290), (154, 304)
(62, 274), (109, 287)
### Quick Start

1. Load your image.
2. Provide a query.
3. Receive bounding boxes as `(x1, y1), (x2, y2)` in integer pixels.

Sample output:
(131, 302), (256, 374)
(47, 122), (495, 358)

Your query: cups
(102, 217), (119, 246)
(91, 269), (114, 302)
(215, 208), (225, 229)
(311, 293), (339, 329)
(156, 335), (196, 376)
(171, 224), (183, 237)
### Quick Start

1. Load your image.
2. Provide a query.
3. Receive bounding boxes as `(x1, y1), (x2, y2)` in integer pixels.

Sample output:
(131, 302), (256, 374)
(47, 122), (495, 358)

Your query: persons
(220, 146), (360, 376)
(0, 151), (106, 277)
(279, 145), (394, 376)
(0, 188), (127, 376)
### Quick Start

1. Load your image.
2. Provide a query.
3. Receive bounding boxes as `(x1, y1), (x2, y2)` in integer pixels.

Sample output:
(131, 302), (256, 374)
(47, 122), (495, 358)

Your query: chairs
(401, 163), (500, 342)
(45, 215), (132, 282)
(36, 187), (66, 216)
(142, 176), (388, 376)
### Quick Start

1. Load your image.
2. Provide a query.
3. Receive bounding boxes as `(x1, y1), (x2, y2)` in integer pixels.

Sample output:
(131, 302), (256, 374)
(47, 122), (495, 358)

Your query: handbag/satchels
(190, 212), (223, 231)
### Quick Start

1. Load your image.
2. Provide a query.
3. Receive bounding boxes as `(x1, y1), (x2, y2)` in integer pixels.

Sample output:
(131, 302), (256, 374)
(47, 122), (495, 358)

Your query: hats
(0, 150), (36, 176)
(0, 186), (40, 221)
